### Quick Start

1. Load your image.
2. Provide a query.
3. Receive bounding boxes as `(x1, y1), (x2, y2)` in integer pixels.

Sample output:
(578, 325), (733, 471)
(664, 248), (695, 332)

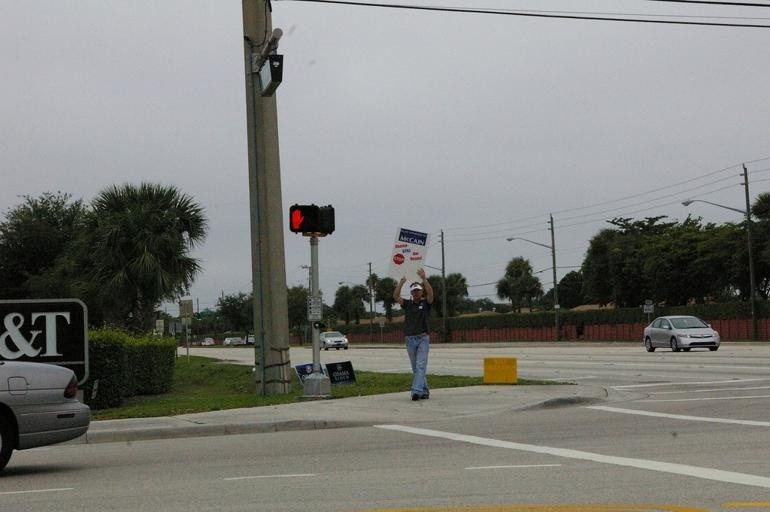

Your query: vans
(201, 337), (216, 346)
(244, 334), (256, 345)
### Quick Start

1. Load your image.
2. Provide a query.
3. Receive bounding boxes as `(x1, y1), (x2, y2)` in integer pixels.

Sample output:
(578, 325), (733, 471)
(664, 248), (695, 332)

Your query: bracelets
(422, 277), (427, 282)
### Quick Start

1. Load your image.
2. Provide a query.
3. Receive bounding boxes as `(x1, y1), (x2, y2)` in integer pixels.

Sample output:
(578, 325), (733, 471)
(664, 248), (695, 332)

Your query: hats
(410, 283), (423, 292)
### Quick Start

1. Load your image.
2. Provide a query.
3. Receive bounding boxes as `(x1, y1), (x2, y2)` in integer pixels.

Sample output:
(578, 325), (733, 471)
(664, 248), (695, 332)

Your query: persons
(393, 267), (434, 400)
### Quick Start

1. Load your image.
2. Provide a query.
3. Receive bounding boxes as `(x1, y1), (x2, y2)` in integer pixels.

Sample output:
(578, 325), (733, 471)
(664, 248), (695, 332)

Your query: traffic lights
(288, 203), (337, 235)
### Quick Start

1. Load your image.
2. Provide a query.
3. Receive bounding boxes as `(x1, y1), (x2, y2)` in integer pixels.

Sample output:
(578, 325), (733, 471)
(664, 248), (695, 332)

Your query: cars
(229, 336), (245, 346)
(643, 313), (721, 353)
(223, 337), (232, 346)
(319, 330), (349, 351)
(0, 356), (91, 469)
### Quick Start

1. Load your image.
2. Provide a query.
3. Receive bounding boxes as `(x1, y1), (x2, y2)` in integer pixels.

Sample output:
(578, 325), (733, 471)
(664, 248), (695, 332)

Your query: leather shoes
(411, 393), (419, 400)
(420, 393), (429, 399)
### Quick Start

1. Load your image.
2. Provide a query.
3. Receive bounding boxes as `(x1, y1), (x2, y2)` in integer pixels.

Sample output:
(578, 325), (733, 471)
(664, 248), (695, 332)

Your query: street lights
(506, 237), (559, 342)
(681, 197), (759, 340)
